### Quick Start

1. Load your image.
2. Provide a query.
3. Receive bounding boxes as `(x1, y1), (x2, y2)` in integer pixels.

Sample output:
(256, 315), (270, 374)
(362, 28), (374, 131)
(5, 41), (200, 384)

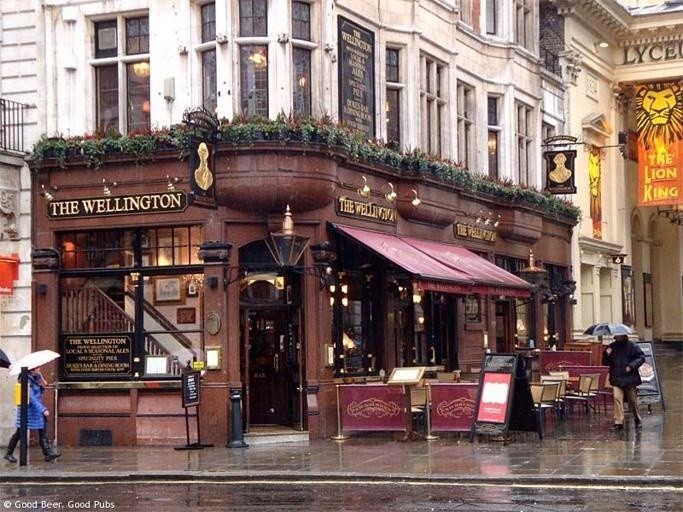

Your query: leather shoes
(610, 424), (623, 432)
(634, 419), (642, 428)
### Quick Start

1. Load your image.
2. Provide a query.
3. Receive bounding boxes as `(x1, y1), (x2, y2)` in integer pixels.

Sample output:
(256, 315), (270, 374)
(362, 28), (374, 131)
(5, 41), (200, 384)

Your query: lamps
(337, 175), (422, 212)
(40, 173), (180, 206)
(263, 203), (311, 267)
(462, 208), (503, 228)
(517, 248), (548, 297)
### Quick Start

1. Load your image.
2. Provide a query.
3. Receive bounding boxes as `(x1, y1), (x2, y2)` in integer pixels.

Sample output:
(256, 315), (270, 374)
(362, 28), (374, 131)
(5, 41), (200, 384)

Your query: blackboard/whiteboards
(182, 373), (200, 408)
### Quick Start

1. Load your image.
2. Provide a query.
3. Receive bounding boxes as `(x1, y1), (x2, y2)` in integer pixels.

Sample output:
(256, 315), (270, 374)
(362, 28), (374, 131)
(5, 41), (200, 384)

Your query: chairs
(406, 368), (600, 438)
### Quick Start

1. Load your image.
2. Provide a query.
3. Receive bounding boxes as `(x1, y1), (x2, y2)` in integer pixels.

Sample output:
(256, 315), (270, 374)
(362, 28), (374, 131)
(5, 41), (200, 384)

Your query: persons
(4, 367), (61, 462)
(602, 335), (646, 431)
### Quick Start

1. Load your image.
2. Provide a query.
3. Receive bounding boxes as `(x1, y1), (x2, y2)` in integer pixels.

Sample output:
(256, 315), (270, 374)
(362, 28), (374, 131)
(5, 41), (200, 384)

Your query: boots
(4, 439), (17, 462)
(40, 439), (61, 461)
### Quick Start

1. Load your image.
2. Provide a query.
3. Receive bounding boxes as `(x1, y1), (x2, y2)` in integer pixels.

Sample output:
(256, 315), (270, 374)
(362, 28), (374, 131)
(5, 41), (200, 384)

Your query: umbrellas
(1, 348), (11, 370)
(583, 323), (637, 349)
(8, 349), (61, 378)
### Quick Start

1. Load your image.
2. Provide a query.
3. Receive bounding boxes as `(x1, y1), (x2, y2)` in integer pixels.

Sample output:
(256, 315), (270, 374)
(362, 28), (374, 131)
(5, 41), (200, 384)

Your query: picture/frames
(140, 252), (152, 286)
(156, 232), (182, 267)
(176, 307), (196, 324)
(139, 230), (150, 250)
(186, 277), (199, 297)
(150, 274), (186, 306)
